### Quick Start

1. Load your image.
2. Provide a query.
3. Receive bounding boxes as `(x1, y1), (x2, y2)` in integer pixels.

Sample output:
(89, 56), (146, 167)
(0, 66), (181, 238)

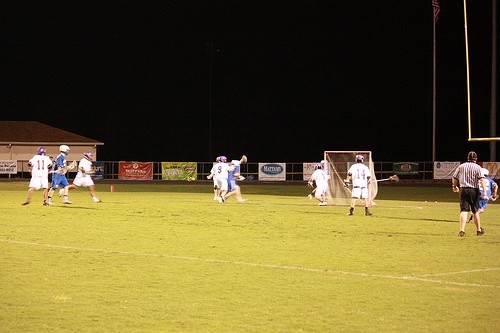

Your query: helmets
(216, 157), (220, 161)
(483, 168), (489, 175)
(314, 163), (322, 170)
(37, 147), (47, 155)
(220, 156), (227, 163)
(467, 151), (478, 162)
(60, 145), (70, 155)
(355, 155), (364, 163)
(84, 152), (93, 160)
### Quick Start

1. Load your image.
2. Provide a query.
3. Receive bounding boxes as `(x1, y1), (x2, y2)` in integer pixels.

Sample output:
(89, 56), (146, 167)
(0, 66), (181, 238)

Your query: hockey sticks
(48, 168), (67, 175)
(232, 155), (247, 167)
(66, 160), (78, 170)
(370, 175), (400, 183)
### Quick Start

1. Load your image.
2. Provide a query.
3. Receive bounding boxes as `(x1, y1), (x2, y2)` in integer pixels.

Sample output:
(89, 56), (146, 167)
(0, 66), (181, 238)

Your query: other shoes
(348, 213), (353, 215)
(220, 196), (225, 204)
(320, 202), (327, 205)
(64, 200), (73, 204)
(93, 198), (102, 203)
(477, 227), (484, 235)
(459, 231), (466, 236)
(366, 213), (372, 216)
(308, 194), (312, 199)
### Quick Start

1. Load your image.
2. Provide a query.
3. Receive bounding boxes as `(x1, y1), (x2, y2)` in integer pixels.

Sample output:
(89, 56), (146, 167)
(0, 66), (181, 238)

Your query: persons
(468, 167), (498, 223)
(58, 152), (102, 203)
(452, 151), (485, 237)
(47, 155), (53, 191)
(308, 164), (328, 206)
(21, 147), (53, 205)
(207, 155), (247, 204)
(346, 155), (372, 216)
(45, 145), (73, 204)
